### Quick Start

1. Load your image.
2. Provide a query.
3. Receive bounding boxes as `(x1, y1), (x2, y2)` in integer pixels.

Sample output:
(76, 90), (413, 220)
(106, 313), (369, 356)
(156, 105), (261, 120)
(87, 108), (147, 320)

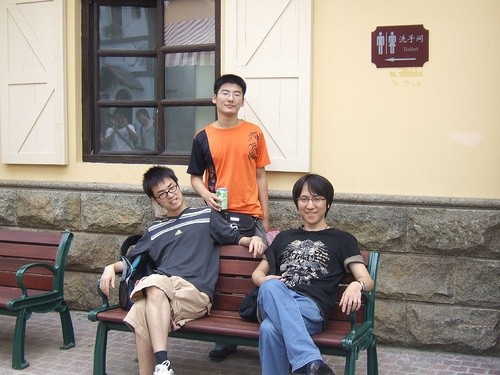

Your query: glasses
(298, 196), (325, 204)
(219, 91), (242, 96)
(155, 183), (177, 199)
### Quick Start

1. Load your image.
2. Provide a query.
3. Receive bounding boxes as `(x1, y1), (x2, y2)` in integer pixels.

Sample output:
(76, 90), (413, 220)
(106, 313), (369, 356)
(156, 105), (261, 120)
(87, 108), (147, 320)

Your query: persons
(251, 175), (373, 375)
(100, 166), (266, 375)
(100, 108), (154, 151)
(187, 74), (270, 360)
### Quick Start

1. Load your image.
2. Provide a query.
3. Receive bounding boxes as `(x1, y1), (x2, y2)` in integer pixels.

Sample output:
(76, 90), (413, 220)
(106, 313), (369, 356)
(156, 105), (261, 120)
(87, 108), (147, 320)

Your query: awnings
(162, 18), (215, 66)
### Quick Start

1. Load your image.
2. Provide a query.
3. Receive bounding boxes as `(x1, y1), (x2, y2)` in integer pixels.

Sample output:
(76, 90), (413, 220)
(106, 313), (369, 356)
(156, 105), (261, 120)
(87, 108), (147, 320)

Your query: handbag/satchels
(238, 286), (259, 323)
(118, 268), (145, 311)
(226, 213), (257, 237)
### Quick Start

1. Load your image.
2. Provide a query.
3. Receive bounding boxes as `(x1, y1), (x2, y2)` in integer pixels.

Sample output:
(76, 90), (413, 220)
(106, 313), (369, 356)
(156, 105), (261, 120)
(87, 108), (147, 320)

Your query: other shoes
(307, 363), (335, 375)
(153, 359), (175, 375)
(209, 344), (237, 361)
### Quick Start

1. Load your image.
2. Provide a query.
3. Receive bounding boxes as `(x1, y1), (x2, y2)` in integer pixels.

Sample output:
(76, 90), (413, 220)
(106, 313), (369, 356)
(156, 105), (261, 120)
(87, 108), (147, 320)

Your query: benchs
(87, 236), (379, 375)
(0, 230), (76, 370)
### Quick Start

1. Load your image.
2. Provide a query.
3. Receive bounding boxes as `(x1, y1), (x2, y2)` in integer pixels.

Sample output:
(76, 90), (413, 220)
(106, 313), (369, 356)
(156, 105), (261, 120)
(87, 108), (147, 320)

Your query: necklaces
(303, 224), (328, 230)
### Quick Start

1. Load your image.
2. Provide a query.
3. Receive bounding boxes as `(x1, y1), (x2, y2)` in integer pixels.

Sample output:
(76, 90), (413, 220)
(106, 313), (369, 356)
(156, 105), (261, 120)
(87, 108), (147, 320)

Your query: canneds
(216, 188), (228, 210)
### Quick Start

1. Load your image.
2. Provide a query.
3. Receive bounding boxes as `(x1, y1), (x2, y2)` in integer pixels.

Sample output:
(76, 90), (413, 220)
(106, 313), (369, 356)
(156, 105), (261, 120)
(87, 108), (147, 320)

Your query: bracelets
(357, 280), (364, 293)
